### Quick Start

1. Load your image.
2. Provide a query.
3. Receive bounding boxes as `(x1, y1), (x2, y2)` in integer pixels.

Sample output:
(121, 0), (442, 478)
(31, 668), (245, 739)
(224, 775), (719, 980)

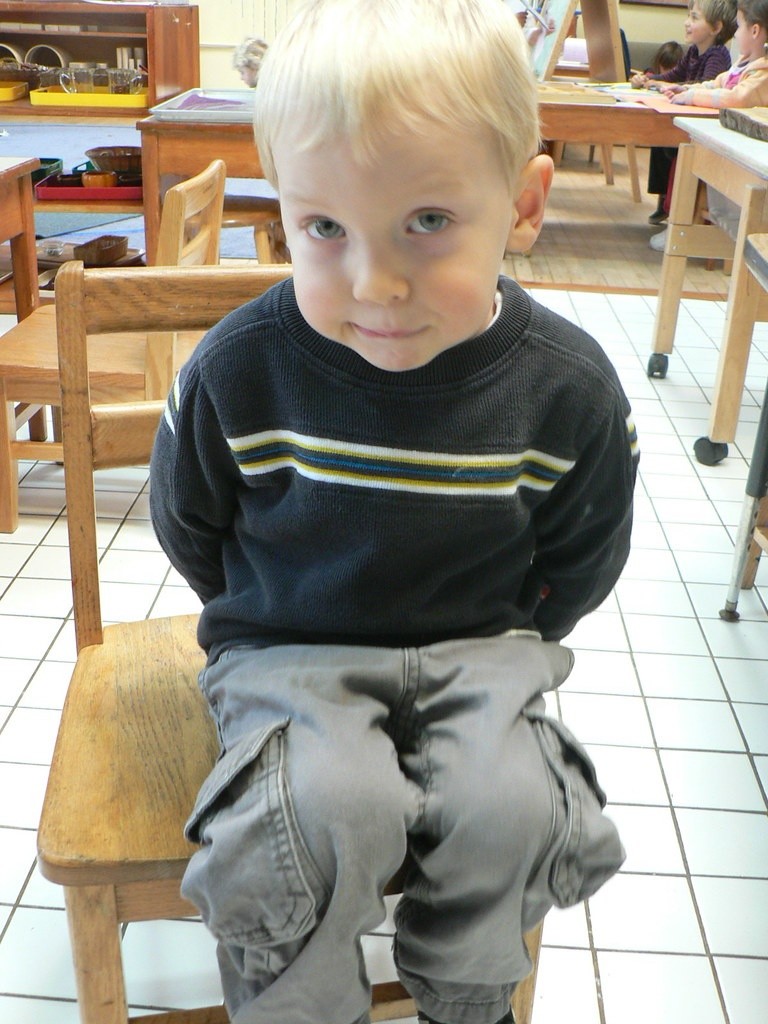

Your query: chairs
(36, 258), (547, 1023)
(0, 158), (227, 533)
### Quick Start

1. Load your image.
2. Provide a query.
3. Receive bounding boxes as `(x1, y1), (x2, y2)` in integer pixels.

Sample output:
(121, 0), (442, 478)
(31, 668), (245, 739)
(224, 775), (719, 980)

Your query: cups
(0, 37), (147, 74)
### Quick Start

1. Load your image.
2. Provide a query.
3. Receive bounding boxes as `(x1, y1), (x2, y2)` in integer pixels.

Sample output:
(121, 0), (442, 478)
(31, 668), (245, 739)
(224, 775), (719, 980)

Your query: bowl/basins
(87, 145), (141, 173)
(37, 239), (65, 256)
(83, 170), (116, 186)
(107, 66), (143, 94)
(58, 68), (94, 94)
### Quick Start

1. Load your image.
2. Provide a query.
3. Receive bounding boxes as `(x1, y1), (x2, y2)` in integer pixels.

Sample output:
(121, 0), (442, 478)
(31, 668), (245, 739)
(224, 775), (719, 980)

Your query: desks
(0, 155), (50, 442)
(135, 76), (718, 265)
(642, 116), (768, 624)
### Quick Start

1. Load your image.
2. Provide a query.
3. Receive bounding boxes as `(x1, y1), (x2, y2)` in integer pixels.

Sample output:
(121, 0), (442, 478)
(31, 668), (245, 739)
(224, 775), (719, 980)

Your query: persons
(509, 7), (555, 52)
(230, 38), (275, 91)
(150, 0), (641, 1024)
(627, 0), (738, 228)
(641, 40), (684, 84)
(648, 1), (768, 252)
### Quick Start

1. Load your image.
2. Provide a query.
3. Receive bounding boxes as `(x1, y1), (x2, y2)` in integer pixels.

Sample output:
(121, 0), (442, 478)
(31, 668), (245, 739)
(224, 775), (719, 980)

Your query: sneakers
(649, 228), (668, 255)
(649, 205), (667, 221)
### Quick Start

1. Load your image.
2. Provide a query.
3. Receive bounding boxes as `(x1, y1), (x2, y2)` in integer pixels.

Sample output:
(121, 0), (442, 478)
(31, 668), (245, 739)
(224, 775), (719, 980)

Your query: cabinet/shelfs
(0, 0), (204, 319)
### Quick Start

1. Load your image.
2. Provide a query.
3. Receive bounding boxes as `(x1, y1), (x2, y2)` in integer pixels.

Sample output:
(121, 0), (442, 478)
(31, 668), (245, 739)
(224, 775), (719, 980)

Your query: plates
(31, 157), (63, 176)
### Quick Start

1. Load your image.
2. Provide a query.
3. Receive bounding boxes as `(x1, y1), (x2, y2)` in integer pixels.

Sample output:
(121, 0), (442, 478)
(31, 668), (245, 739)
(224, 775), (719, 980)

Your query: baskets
(86, 144), (145, 175)
(74, 236), (127, 264)
